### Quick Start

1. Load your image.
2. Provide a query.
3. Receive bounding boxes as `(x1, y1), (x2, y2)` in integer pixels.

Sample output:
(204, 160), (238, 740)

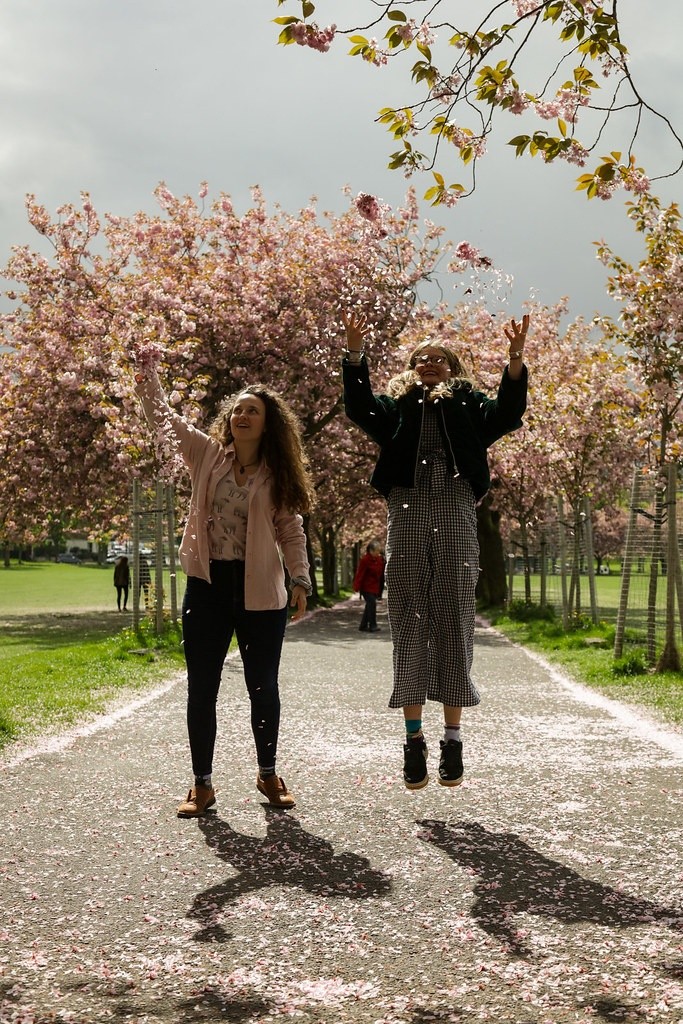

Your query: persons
(353, 540), (386, 632)
(134, 356), (318, 818)
(337, 309), (530, 789)
(139, 553), (153, 611)
(113, 556), (130, 612)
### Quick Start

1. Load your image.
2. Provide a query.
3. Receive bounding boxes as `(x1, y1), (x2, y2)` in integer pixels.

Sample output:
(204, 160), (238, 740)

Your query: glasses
(415, 354), (446, 367)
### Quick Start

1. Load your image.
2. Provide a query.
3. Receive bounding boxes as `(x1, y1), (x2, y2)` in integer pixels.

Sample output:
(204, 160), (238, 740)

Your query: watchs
(346, 351), (361, 361)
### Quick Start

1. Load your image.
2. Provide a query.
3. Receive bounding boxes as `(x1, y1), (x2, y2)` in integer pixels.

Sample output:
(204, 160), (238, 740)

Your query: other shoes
(366, 626), (380, 632)
(177, 787), (216, 816)
(256, 774), (296, 806)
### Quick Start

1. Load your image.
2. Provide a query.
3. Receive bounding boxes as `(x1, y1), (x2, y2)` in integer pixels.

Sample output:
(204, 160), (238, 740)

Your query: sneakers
(438, 739), (464, 787)
(403, 734), (429, 790)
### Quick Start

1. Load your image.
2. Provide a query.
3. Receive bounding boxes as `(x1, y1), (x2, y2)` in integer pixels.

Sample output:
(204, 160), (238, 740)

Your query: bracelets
(290, 576), (312, 595)
(510, 349), (524, 360)
